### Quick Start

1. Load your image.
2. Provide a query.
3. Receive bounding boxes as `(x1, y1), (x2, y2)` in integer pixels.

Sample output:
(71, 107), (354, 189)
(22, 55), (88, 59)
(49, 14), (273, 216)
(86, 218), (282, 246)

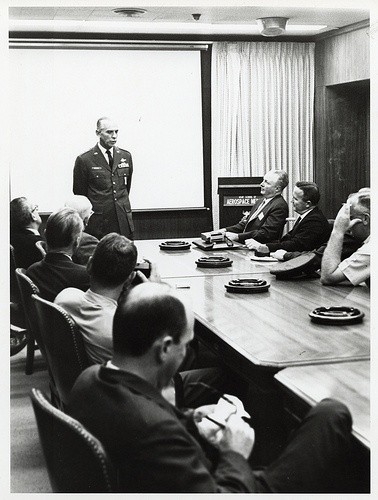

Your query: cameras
(201, 230), (226, 243)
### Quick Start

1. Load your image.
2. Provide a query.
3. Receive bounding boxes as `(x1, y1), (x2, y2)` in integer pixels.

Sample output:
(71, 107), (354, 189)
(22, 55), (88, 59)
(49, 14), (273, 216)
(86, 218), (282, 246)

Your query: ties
(106, 150), (114, 170)
(292, 216), (301, 228)
(248, 200), (265, 223)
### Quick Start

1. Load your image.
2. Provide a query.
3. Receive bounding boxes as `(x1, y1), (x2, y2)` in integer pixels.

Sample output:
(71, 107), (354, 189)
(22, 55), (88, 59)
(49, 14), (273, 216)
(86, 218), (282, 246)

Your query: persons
(9, 170), (364, 409)
(320, 187), (371, 289)
(73, 117), (135, 240)
(65, 281), (352, 494)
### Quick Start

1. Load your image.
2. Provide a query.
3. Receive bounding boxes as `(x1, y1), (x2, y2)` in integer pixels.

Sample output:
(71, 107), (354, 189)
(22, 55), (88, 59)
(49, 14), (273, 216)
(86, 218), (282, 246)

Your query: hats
(270, 252), (321, 281)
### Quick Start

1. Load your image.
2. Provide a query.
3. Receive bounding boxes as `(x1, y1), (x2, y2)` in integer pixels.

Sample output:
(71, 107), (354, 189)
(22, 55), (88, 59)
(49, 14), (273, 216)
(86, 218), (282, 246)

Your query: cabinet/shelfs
(219, 176), (263, 230)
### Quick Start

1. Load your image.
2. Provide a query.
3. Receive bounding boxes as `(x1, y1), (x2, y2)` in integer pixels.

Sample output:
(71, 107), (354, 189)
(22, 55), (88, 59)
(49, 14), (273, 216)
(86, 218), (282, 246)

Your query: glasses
(31, 205), (39, 214)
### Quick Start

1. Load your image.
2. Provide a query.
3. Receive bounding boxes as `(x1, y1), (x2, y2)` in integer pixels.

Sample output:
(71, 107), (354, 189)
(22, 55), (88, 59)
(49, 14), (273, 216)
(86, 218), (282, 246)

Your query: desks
(131, 234), (370, 452)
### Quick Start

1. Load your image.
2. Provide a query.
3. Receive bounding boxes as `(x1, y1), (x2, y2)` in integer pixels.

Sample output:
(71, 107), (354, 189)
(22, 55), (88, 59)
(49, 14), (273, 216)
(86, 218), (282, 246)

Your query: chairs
(10, 245), (40, 376)
(28, 386), (113, 494)
(29, 294), (91, 411)
